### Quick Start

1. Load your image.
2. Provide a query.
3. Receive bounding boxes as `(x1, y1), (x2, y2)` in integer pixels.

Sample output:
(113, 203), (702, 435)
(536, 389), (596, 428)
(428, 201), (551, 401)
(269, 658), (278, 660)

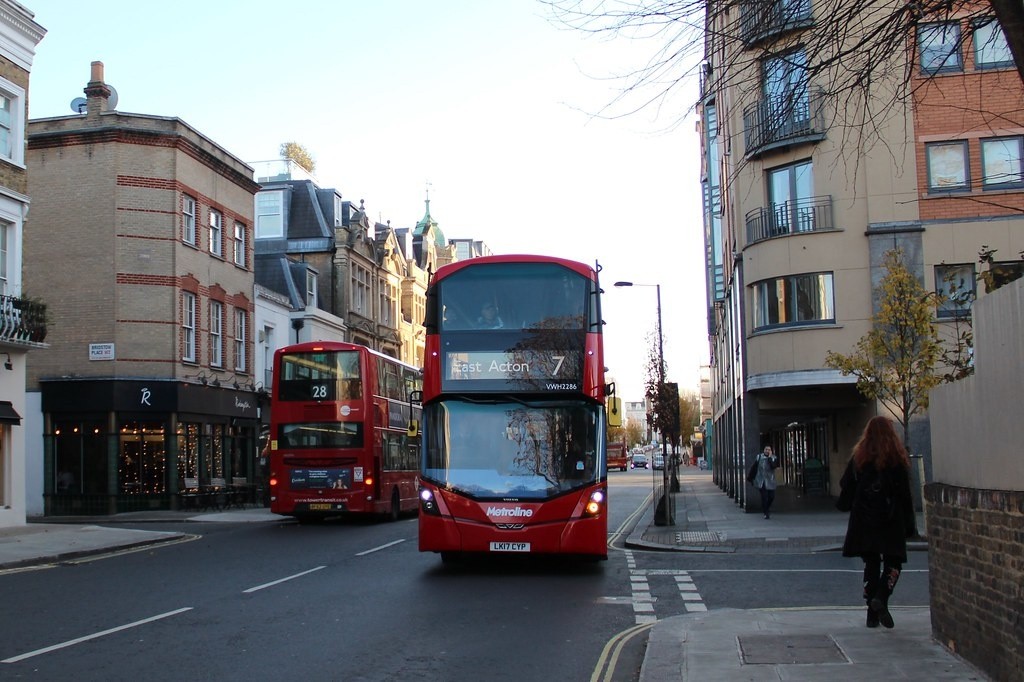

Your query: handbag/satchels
(748, 453), (761, 487)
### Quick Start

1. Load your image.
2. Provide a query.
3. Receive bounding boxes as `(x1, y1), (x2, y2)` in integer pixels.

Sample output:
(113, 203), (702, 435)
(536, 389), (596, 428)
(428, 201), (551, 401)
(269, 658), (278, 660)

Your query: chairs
(551, 433), (583, 479)
(464, 303), (485, 327)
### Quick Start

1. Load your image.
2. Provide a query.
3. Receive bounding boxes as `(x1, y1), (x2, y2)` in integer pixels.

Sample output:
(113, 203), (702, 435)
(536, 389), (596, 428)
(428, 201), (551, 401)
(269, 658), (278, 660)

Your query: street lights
(613, 281), (672, 527)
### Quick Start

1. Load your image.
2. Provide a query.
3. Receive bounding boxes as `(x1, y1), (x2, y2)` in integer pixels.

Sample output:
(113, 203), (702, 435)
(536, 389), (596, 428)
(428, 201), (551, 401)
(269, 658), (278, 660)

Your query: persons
(753, 445), (778, 519)
(443, 305), (460, 330)
(474, 302), (504, 329)
(839, 416), (923, 629)
(683, 452), (689, 466)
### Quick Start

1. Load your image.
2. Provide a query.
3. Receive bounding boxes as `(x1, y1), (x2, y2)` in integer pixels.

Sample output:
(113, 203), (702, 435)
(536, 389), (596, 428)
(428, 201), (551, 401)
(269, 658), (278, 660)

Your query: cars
(631, 454), (649, 469)
(626, 440), (670, 470)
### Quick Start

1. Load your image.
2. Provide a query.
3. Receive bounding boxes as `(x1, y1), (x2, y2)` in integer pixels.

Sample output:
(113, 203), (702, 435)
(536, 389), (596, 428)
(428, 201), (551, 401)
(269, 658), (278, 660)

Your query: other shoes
(761, 512), (769, 519)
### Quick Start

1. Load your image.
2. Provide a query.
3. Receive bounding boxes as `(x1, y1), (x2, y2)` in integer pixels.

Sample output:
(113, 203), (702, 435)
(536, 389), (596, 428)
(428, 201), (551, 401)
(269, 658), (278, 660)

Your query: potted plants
(0, 292), (56, 342)
(279, 141), (316, 180)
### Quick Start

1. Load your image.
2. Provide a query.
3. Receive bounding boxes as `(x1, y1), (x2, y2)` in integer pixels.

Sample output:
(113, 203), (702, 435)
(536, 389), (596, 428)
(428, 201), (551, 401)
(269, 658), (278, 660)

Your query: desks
(201, 484), (224, 513)
(227, 484), (260, 510)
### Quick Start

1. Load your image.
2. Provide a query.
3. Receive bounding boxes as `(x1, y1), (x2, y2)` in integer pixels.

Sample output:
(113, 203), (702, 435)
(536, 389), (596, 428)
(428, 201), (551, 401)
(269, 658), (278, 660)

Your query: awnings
(0, 402), (22, 426)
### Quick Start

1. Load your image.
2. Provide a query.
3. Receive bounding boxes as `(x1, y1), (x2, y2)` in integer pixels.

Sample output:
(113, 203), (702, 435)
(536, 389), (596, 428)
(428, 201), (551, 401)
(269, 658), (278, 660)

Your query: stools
(184, 478), (200, 512)
(211, 478), (226, 510)
(232, 477), (247, 508)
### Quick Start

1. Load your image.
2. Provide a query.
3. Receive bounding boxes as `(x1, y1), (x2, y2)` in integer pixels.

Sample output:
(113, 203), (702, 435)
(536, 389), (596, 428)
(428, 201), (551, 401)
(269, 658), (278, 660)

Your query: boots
(869, 567), (903, 628)
(862, 569), (881, 628)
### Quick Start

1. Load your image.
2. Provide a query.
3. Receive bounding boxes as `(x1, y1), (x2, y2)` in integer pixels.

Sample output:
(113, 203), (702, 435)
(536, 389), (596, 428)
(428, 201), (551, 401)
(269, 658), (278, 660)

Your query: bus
(270, 340), (425, 523)
(408, 253), (621, 570)
(605, 442), (628, 472)
